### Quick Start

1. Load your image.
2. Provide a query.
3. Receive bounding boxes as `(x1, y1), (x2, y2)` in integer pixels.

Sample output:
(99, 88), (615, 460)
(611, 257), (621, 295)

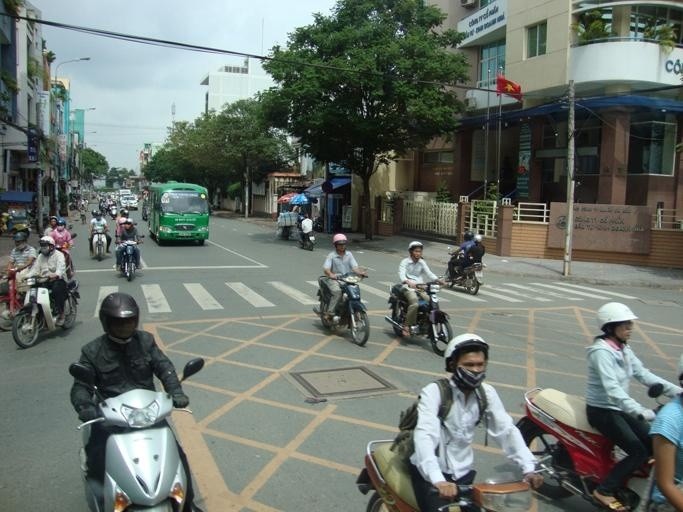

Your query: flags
(497, 71), (522, 100)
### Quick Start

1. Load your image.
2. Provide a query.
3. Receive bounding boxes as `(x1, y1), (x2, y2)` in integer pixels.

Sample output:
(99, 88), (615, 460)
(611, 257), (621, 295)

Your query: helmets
(443, 333), (489, 370)
(92, 210), (101, 216)
(332, 234), (348, 245)
(408, 241), (423, 252)
(597, 302), (637, 330)
(464, 231), (481, 241)
(13, 232), (27, 241)
(39, 236), (55, 246)
(118, 208), (133, 224)
(50, 215), (66, 225)
(99, 294), (140, 338)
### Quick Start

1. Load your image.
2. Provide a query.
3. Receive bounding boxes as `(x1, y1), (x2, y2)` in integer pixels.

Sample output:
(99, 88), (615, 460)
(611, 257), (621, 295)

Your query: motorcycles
(0, 209), (32, 241)
(355, 434), (554, 511)
(88, 189), (145, 281)
(311, 272), (371, 346)
(383, 276), (454, 357)
(444, 251), (487, 294)
(512, 372), (682, 512)
(67, 357), (204, 512)
(296, 229), (316, 250)
(0, 233), (80, 347)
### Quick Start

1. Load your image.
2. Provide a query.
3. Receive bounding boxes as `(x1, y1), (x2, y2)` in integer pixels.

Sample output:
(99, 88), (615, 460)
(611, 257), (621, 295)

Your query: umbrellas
(278, 192), (300, 204)
(289, 193), (318, 212)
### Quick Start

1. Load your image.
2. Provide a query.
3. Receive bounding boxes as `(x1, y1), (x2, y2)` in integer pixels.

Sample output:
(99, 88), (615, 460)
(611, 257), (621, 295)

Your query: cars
(140, 201), (150, 220)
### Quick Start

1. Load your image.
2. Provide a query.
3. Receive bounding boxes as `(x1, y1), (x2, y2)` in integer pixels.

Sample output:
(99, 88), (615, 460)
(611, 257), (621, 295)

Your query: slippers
(591, 492), (631, 512)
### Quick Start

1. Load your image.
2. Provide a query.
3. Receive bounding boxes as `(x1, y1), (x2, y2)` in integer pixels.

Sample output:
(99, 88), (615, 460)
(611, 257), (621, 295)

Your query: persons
(288, 205), (294, 212)
(5, 194), (140, 279)
(292, 205), (299, 212)
(587, 302), (682, 512)
(299, 205), (307, 216)
(407, 333), (544, 512)
(398, 241), (447, 337)
(297, 212), (305, 242)
(455, 235), (485, 280)
(70, 293), (194, 511)
(300, 214), (313, 243)
(24, 236), (69, 327)
(322, 234), (367, 325)
(648, 372), (683, 512)
(451, 231), (475, 280)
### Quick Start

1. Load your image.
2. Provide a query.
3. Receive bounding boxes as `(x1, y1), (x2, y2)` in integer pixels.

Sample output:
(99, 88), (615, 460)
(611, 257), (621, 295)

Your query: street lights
(84, 130), (96, 150)
(170, 100), (176, 131)
(69, 107), (96, 182)
(54, 56), (92, 217)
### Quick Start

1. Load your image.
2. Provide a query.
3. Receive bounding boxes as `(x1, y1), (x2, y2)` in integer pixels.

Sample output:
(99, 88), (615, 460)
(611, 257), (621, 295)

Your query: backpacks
(389, 380), (488, 459)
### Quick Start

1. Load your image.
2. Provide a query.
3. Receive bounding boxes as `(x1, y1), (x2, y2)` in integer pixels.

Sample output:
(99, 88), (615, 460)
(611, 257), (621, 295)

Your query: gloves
(172, 393), (188, 408)
(78, 404), (97, 421)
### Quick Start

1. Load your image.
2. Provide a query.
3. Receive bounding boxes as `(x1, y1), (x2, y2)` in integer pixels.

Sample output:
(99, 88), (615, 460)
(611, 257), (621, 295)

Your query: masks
(455, 366), (487, 391)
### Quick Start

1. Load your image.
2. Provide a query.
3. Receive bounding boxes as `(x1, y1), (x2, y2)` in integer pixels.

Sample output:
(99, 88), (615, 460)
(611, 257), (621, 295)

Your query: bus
(145, 181), (211, 246)
